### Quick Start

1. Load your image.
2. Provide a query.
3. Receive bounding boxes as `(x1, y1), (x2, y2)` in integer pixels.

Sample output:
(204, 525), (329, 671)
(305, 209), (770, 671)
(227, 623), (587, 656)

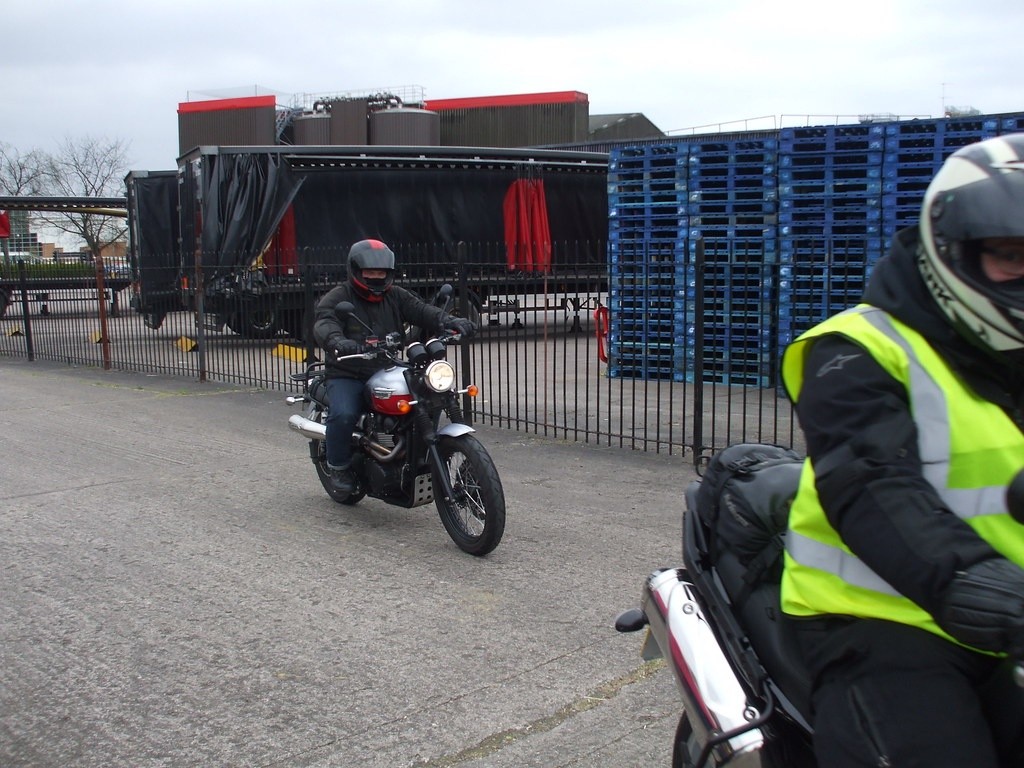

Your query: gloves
(326, 336), (367, 355)
(944, 557), (1024, 658)
(443, 315), (478, 339)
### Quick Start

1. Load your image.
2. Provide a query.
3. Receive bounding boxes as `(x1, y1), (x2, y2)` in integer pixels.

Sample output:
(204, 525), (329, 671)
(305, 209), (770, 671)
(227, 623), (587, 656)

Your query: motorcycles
(615, 443), (1024, 768)
(285, 284), (505, 559)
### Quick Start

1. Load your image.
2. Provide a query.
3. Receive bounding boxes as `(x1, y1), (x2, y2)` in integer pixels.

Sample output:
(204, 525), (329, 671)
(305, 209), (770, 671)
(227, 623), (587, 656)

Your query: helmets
(347, 239), (395, 303)
(915, 132), (1024, 360)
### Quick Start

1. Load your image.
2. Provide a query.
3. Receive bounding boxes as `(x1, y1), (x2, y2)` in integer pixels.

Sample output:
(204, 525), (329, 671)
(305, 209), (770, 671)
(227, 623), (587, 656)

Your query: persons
(311, 238), (480, 498)
(780, 132), (1024, 765)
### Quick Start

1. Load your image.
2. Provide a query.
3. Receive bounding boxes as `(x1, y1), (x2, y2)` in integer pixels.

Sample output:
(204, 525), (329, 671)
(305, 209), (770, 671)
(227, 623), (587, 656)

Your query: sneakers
(328, 463), (357, 492)
